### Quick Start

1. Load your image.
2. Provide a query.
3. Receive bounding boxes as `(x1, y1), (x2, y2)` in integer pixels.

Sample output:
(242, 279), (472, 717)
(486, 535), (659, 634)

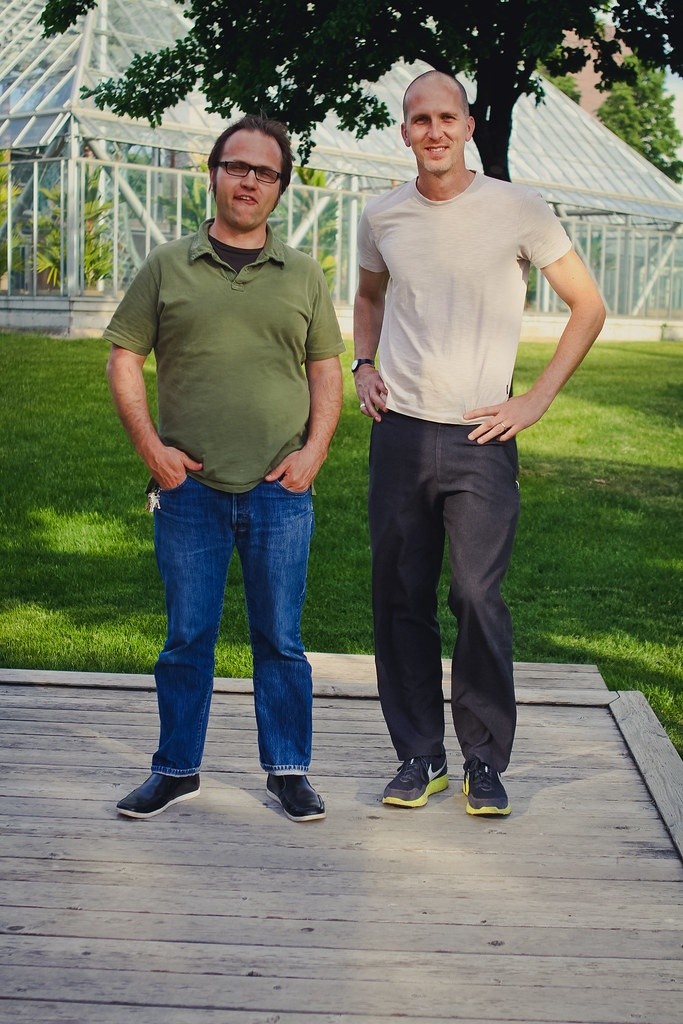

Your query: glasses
(218, 161), (282, 184)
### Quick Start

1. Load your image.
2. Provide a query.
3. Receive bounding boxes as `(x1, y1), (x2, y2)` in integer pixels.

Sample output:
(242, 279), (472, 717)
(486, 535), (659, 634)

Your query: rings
(360, 404), (366, 408)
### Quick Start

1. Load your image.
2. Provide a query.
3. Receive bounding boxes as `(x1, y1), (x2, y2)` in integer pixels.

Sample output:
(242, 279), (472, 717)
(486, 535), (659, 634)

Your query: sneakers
(383, 754), (448, 807)
(462, 761), (511, 815)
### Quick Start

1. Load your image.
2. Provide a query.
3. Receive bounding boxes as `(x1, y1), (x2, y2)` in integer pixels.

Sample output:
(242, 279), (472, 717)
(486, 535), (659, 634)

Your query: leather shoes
(266, 773), (326, 821)
(117, 773), (200, 818)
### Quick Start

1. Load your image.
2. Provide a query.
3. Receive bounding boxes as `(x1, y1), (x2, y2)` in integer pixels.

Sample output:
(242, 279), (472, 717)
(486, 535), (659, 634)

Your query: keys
(146, 488), (161, 513)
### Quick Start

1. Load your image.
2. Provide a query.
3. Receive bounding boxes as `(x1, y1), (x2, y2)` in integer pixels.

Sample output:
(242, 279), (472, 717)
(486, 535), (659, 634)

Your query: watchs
(351, 358), (374, 372)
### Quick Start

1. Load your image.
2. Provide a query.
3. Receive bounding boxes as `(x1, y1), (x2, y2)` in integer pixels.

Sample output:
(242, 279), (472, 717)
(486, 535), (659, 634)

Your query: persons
(354, 65), (609, 818)
(103, 114), (344, 823)
